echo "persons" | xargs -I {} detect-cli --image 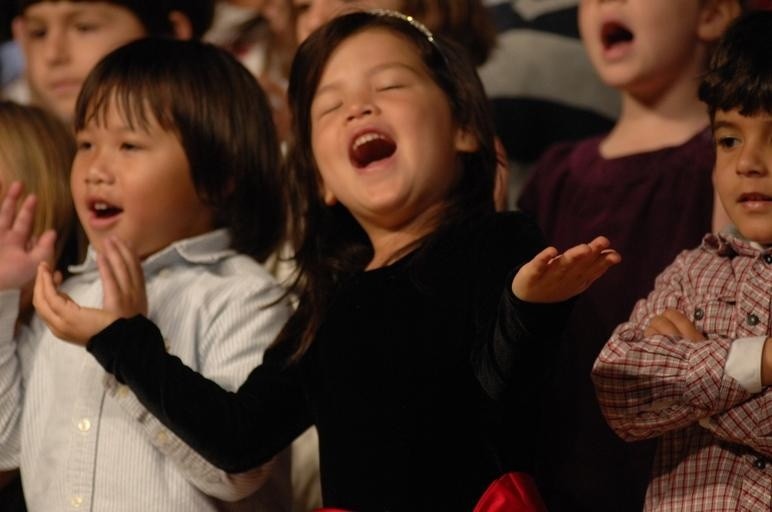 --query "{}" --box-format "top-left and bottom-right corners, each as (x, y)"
(0, 1), (771, 511)
